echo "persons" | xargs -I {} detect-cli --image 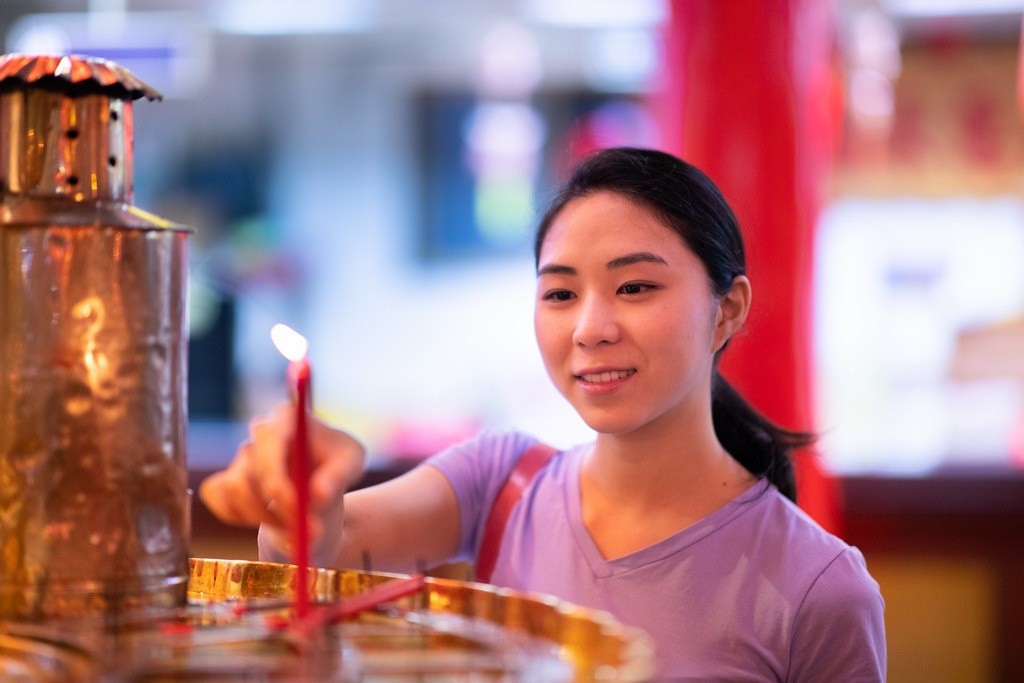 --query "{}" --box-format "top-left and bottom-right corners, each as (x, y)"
(199, 146), (890, 683)
